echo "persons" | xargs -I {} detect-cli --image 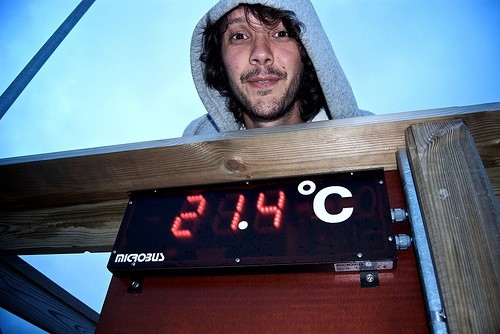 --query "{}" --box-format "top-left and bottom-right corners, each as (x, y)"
(180, 0), (373, 136)
